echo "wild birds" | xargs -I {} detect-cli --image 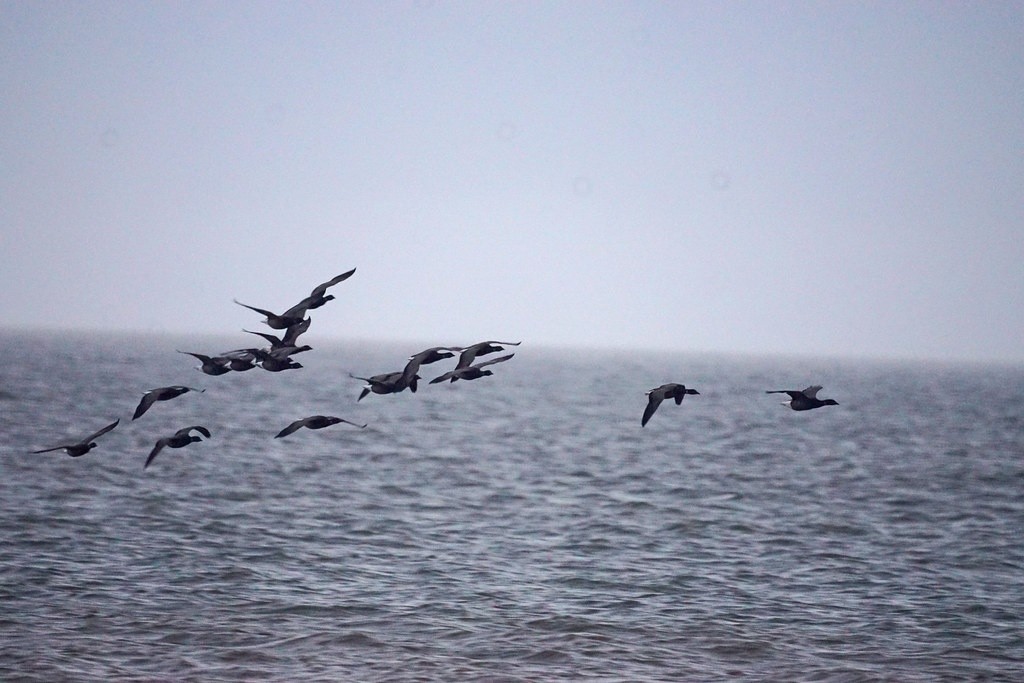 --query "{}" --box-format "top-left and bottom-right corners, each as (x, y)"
(131, 385), (206, 421)
(177, 267), (357, 376)
(351, 341), (522, 403)
(33, 418), (120, 457)
(144, 425), (211, 470)
(274, 416), (368, 439)
(766, 385), (840, 411)
(641, 383), (700, 427)
